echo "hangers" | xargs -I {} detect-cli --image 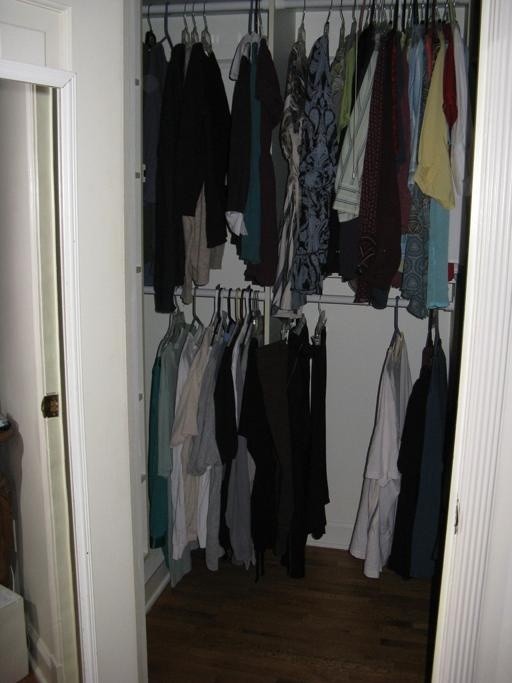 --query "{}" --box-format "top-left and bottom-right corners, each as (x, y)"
(157, 286), (440, 359)
